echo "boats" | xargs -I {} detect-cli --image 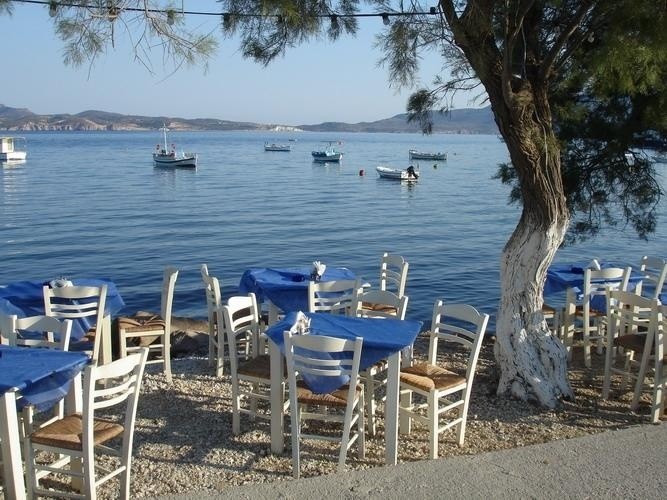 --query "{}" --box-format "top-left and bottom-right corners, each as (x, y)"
(0, 136), (28, 161)
(288, 137), (297, 143)
(496, 133), (504, 143)
(151, 121), (199, 169)
(624, 147), (646, 172)
(375, 165), (420, 181)
(408, 148), (448, 162)
(650, 153), (666, 164)
(261, 142), (292, 153)
(309, 147), (344, 163)
